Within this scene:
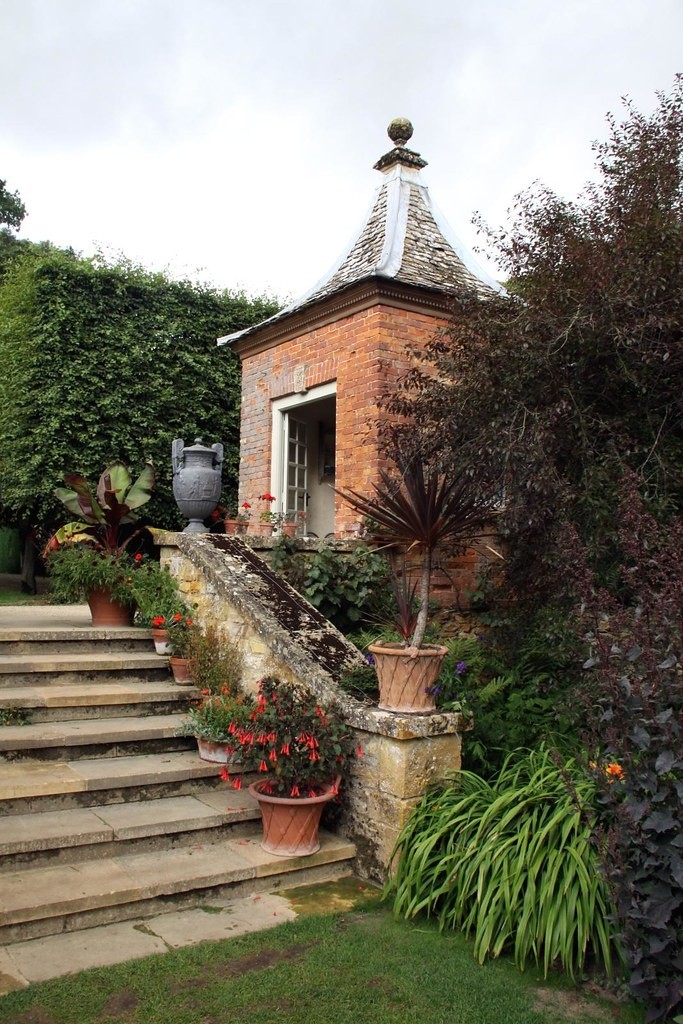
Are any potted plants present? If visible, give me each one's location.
[423,595,430,597]
[42,460,170,627]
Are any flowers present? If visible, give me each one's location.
[213,494,253,520]
[172,679,259,738]
[277,511,305,523]
[146,593,193,628]
[217,677,363,798]
[257,493,277,522]
[166,624,204,657]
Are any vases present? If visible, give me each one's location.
[259,522,275,536]
[327,438,502,713]
[151,628,175,656]
[283,523,296,537]
[195,736,245,763]
[247,778,339,857]
[225,520,250,535]
[170,657,195,685]
